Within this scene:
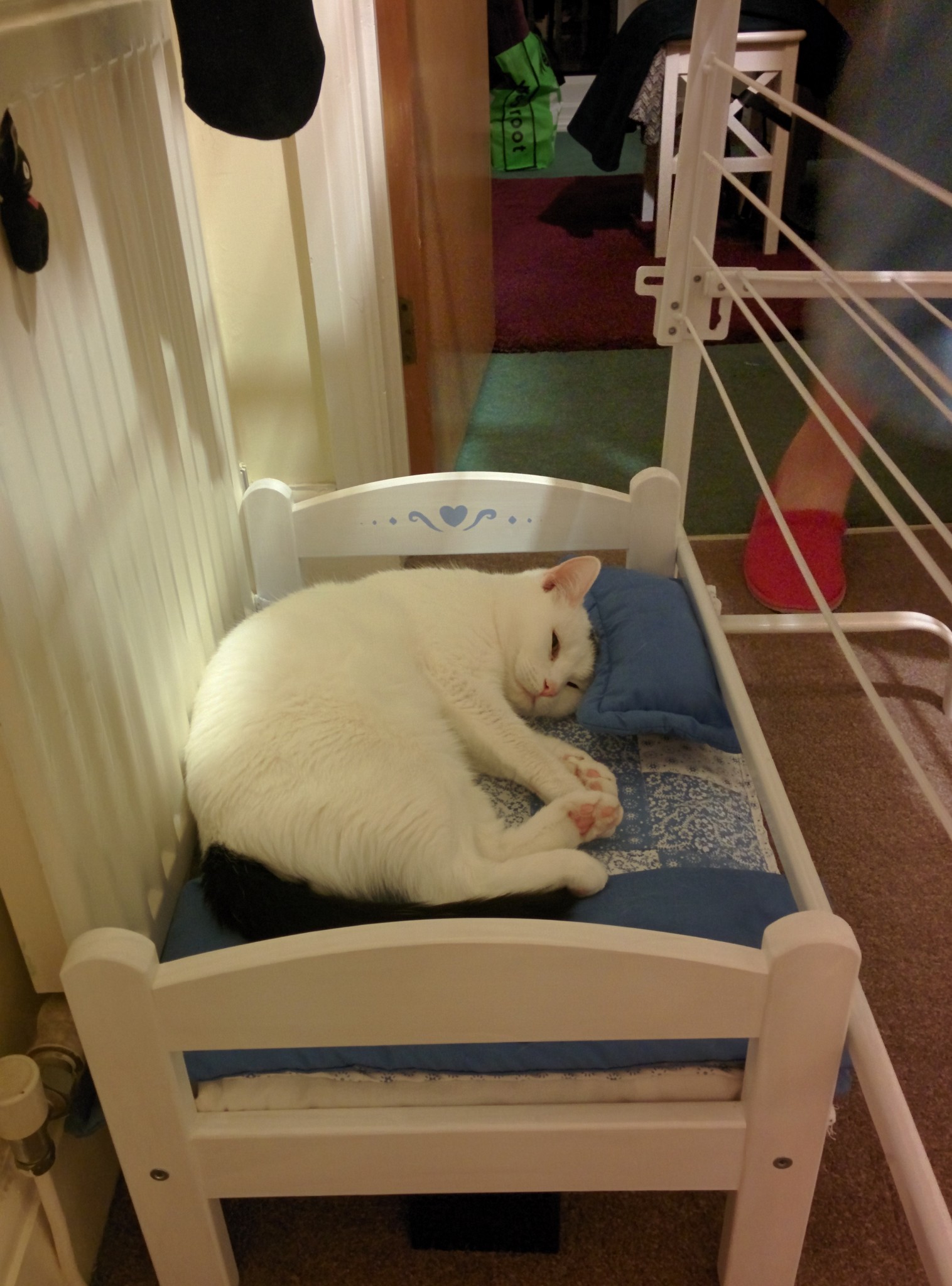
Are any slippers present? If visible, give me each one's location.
[742,485,845,615]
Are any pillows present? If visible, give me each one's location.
[579,556,743,758]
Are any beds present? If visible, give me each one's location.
[52,466,864,1286]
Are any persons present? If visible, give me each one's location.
[743,0,952,613]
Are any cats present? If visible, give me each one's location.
[186,555,624,944]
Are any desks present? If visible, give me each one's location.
[644,26,807,257]
